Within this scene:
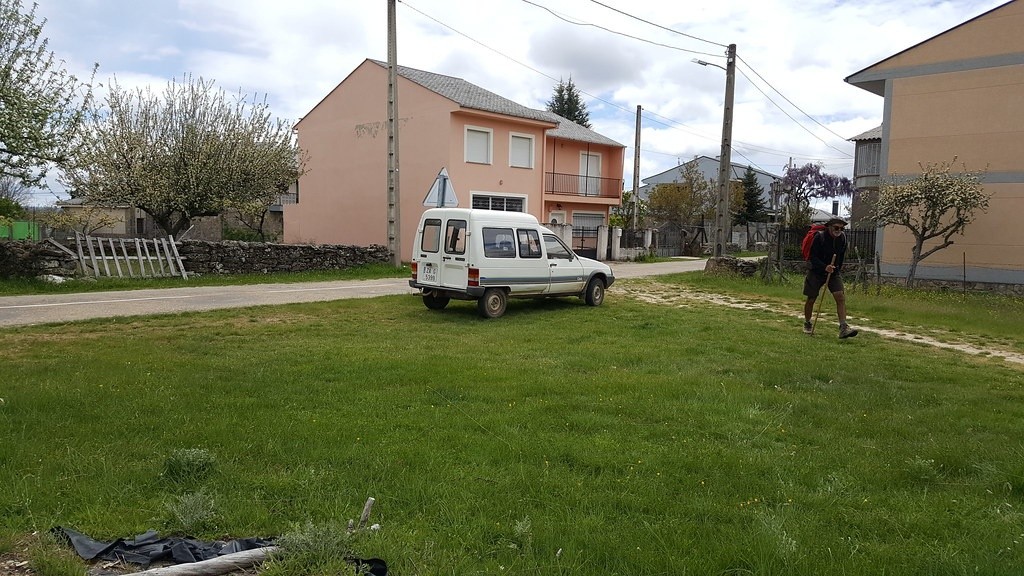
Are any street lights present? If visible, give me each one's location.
[690,44,736,256]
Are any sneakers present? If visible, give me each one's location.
[803,321,813,334]
[838,323,858,339]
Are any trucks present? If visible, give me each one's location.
[409,208,615,318]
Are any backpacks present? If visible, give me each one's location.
[801,225,826,268]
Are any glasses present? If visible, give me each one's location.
[834,226,845,231]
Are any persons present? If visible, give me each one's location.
[802,216,858,338]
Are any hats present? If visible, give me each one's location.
[824,216,848,227]
